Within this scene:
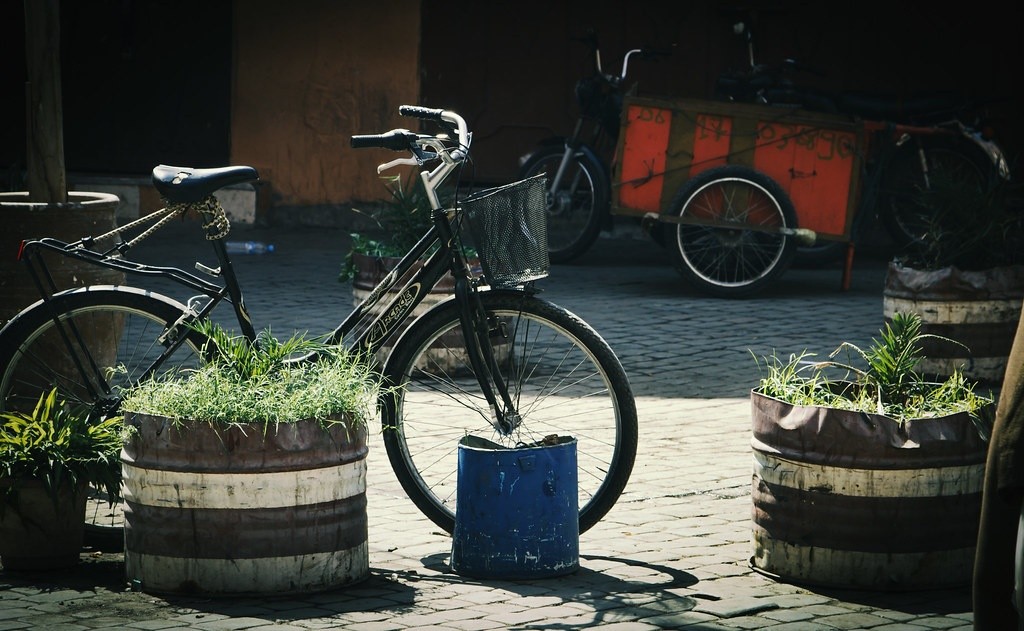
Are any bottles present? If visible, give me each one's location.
[225,241,275,256]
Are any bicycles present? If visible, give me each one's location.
[0,105,639,551]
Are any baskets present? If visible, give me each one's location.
[457,172,551,286]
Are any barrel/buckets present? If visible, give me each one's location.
[451,434,580,580]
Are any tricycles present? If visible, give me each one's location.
[509,41,871,298]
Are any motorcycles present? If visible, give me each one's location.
[713,21,1012,269]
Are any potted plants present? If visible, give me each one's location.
[746,309,999,595]
[0,385,124,573]
[882,150,1024,389]
[102,314,415,605]
[334,165,518,379]
